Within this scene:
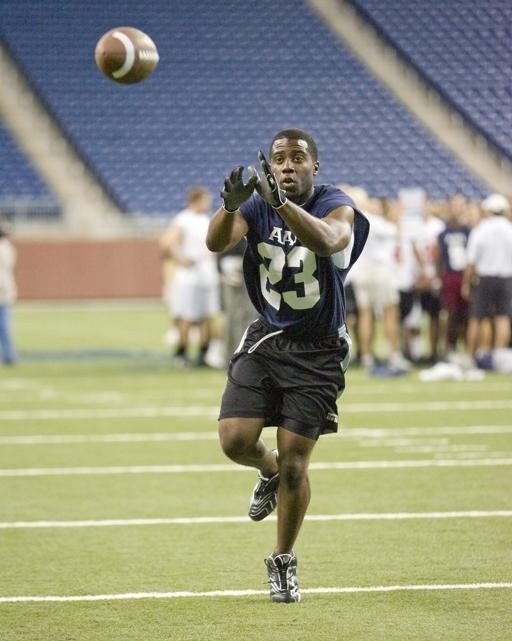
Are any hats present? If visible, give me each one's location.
[481,193,509,211]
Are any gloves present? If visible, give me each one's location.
[219,151,287,213]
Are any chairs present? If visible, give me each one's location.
[0,0,512,215]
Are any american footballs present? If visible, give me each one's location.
[95,26,160,85]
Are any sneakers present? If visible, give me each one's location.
[357,348,512,382]
[172,347,227,370]
[248,448,280,521]
[263,549,301,602]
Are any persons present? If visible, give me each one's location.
[205,128,369,603]
[334,182,512,379]
[0,216,21,364]
[156,188,259,370]
